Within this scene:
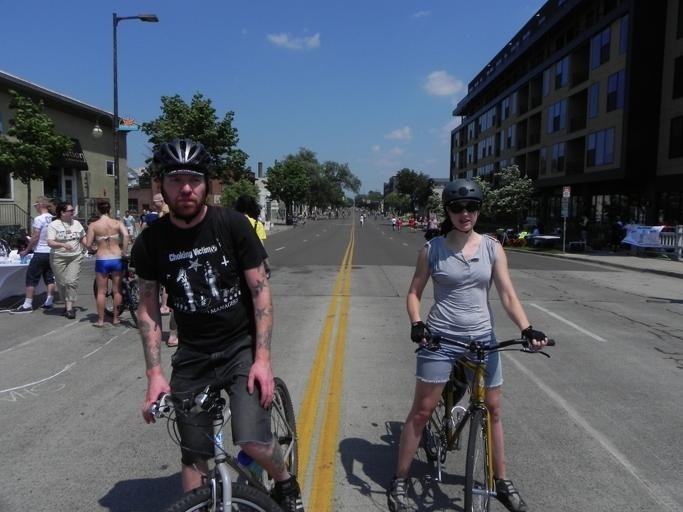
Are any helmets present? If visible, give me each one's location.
[153,138,210,177]
[442,179,482,206]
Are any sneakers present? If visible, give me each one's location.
[387,477,409,512]
[275,472,304,512]
[40,304,53,311]
[493,476,528,512]
[64,310,75,318]
[10,305,32,313]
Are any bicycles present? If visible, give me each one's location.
[146,376,298,512]
[93,257,140,330]
[416,324,554,512]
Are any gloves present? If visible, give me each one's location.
[411,321,430,343]
[521,325,546,348]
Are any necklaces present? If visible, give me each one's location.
[61,219,73,234]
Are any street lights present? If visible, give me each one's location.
[112,12,160,222]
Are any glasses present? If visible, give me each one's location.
[450,202,478,213]
[65,209,74,212]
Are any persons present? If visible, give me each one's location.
[363,213,368,230]
[139,209,147,230]
[528,228,532,234]
[610,215,635,238]
[46,196,63,220]
[84,199,129,327]
[392,216,396,231]
[153,192,169,217]
[359,213,364,231]
[579,215,590,250]
[146,208,158,227]
[537,222,545,234]
[46,204,95,320]
[408,216,440,232]
[388,179,548,512]
[527,225,540,248]
[122,210,135,243]
[8,195,56,313]
[130,137,306,512]
[235,194,266,248]
[398,218,402,231]
